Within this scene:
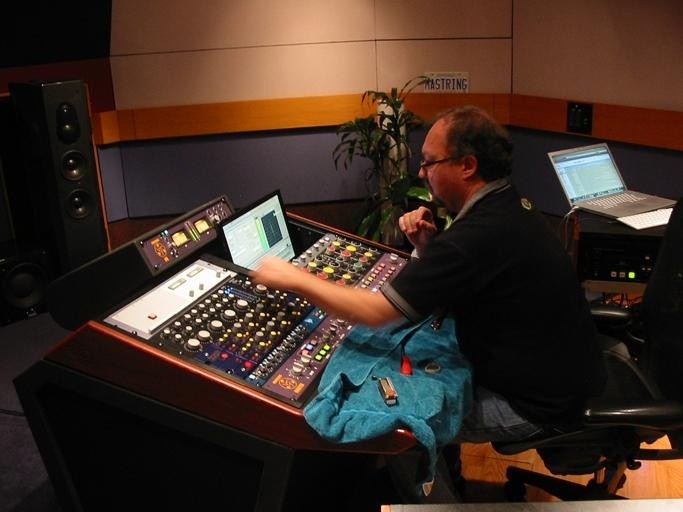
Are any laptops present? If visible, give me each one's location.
[547,142,678,221]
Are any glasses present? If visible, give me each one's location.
[420,156,464,167]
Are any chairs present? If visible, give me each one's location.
[502,198,683,501]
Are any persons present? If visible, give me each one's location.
[248,107,607,443]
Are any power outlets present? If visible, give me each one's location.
[566,101,593,136]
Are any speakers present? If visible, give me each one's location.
[7,78,112,279]
[0,249,58,326]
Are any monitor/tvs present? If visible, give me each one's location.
[218,188,297,275]
